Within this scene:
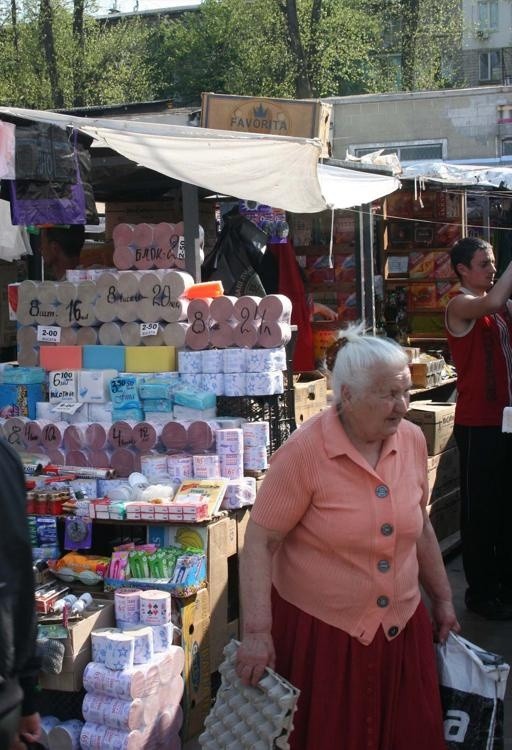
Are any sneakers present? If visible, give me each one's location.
[465,582,512,621]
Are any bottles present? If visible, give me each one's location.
[27,489,70,516]
[48,593,92,615]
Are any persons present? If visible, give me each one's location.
[233,321,462,750]
[440,234,512,621]
[34,223,86,281]
[312,301,339,322]
[0,436,45,749]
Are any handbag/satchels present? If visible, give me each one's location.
[434,629,511,749]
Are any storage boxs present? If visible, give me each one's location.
[201,91,332,163]
[36,598,116,692]
[105,200,217,246]
[162,590,214,749]
[422,489,464,541]
[407,403,459,455]
[421,447,463,505]
[293,370,329,430]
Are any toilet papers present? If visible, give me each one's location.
[33,587,186,749]
[0,222,292,519]
